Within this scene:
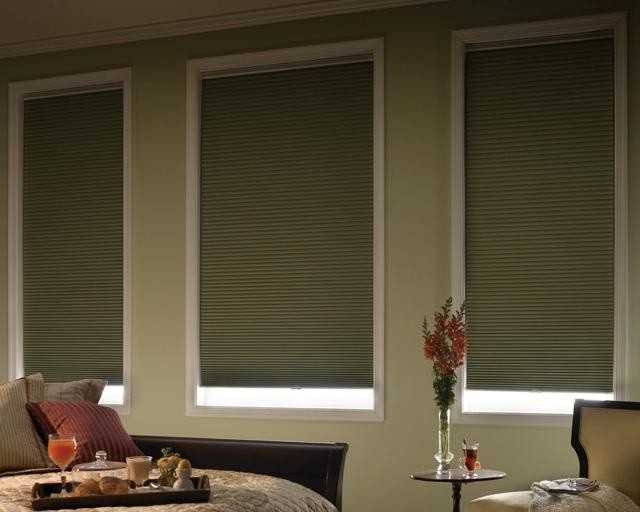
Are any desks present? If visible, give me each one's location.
[409,468,506,512]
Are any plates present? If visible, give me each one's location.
[30,475,210,511]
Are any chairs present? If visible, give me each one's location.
[469,397,640,512]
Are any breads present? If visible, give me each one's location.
[74,478,99,496]
[99,476,129,495]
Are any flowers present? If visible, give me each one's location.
[419,298,473,407]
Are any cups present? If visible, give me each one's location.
[463,444,478,477]
[48,434,76,497]
[127,456,153,490]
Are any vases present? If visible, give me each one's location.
[434,408,454,477]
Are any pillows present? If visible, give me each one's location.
[0,372,151,475]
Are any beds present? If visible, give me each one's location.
[0,435,349,512]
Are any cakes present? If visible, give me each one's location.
[156,447,182,487]
